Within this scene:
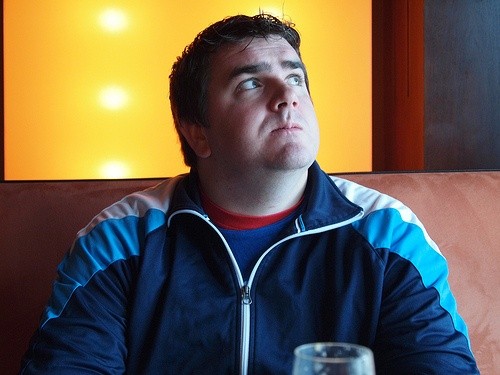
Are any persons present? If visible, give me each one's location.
[18,15,480,375]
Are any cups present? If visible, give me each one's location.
[292,342,376,375]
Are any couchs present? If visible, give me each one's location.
[0,168,500,375]
